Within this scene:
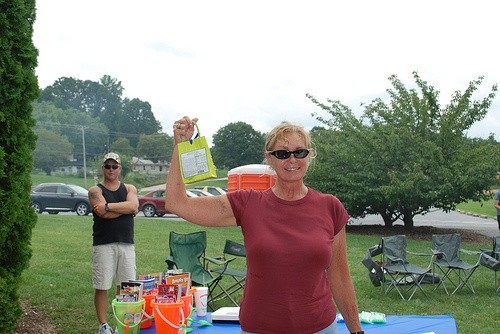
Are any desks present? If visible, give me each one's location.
[114,310,457,334]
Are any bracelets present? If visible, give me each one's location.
[351,331,364,334]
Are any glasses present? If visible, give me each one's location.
[105,164,119,170]
[269,148,310,160]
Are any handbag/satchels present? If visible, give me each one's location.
[177,121,217,184]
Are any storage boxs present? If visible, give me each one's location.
[211,306,240,324]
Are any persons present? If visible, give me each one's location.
[164,115,366,334]
[87,152,140,334]
[494,189,500,230]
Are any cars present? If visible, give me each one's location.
[138,189,199,218]
[188,185,229,196]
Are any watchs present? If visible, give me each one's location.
[104,203,110,212]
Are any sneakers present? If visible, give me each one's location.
[99,323,112,334]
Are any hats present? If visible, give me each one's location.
[103,153,120,164]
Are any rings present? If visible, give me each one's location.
[176,123,181,128]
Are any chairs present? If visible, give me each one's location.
[363,232,500,301]
[164,230,247,312]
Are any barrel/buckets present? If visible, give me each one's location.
[111,287,194,334]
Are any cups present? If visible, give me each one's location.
[194,287,209,317]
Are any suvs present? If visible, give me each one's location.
[29,182,94,216]
